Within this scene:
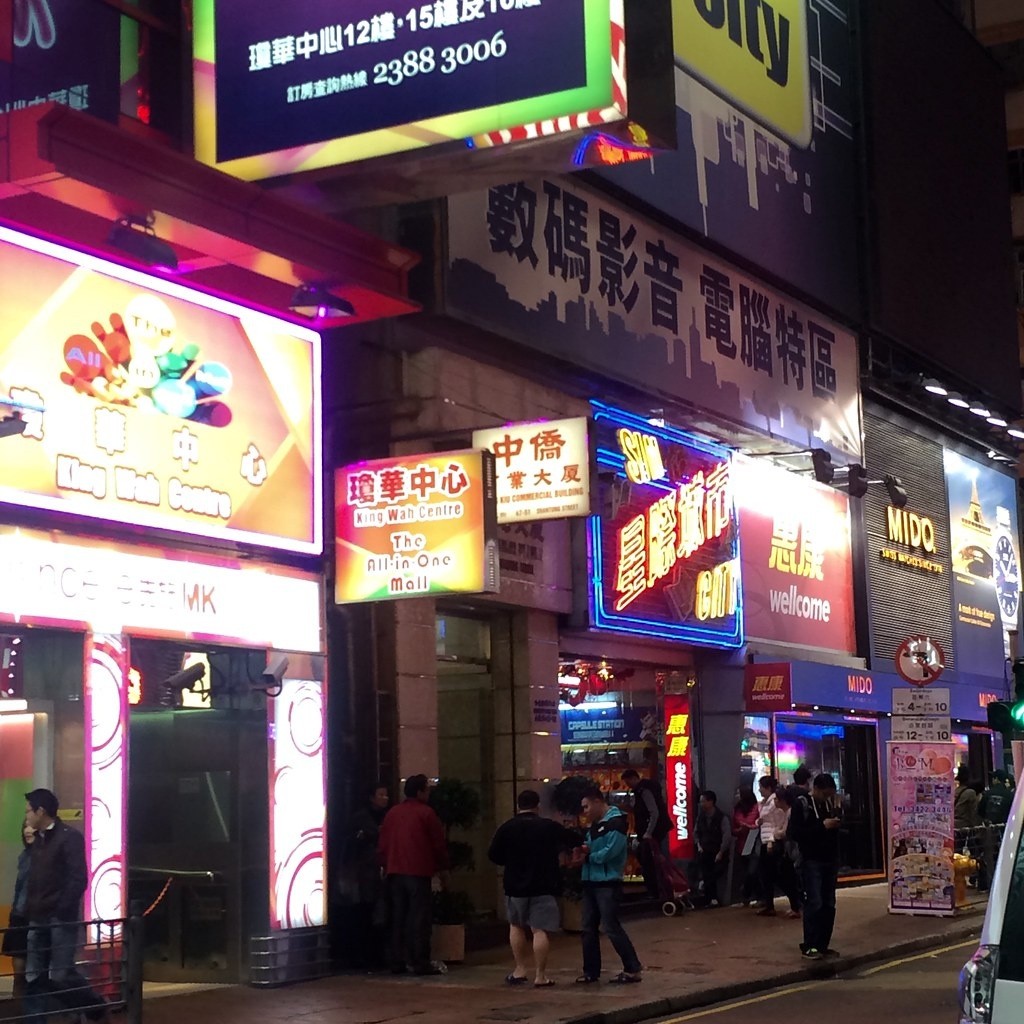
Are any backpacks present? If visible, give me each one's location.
[783,796,833,868]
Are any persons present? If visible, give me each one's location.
[618,769,814,920]
[337,782,399,976]
[488,790,576,987]
[572,789,644,985]
[789,774,849,962]
[955,766,1016,896]
[374,774,451,978]
[1,818,85,1024]
[23,788,112,1024]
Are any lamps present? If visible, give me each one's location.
[833,474,908,508]
[106,214,178,271]
[969,401,991,417]
[744,448,834,484]
[921,377,948,396]
[947,391,970,408]
[289,285,354,319]
[986,411,1008,427]
[1007,424,1024,439]
[791,463,869,499]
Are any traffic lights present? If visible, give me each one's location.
[1012,657,1024,699]
[986,700,1024,733]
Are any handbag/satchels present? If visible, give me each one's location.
[741,826,761,856]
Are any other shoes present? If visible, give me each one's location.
[415,965,442,975]
[783,910,801,918]
[752,900,765,909]
[817,948,839,958]
[730,901,745,908]
[755,907,776,917]
[705,899,719,909]
[696,881,704,896]
[801,948,823,959]
[392,962,408,973]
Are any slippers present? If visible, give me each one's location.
[609,972,642,983]
[505,971,529,984]
[534,978,556,987]
[576,974,599,984]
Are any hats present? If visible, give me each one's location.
[954,766,977,780]
[989,769,1006,780]
